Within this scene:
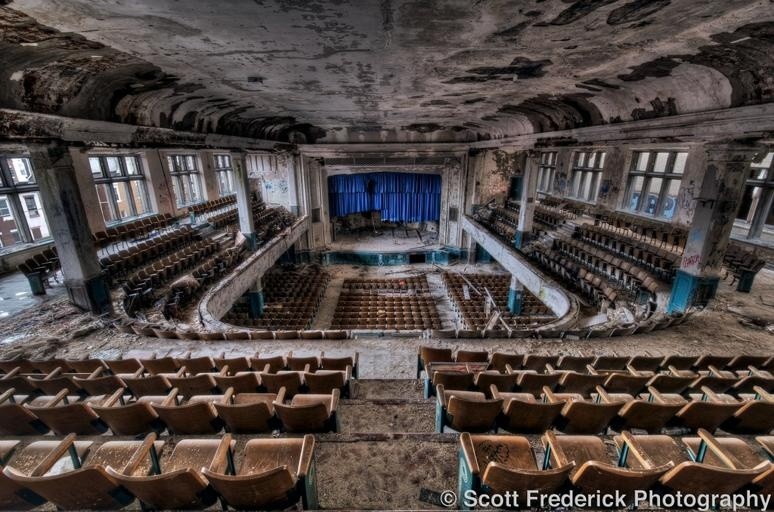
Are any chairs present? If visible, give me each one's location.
[2,322,354,511]
[19,181,294,321]
[411,321,774,510]
[294,258,488,328]
[490,185,763,319]
